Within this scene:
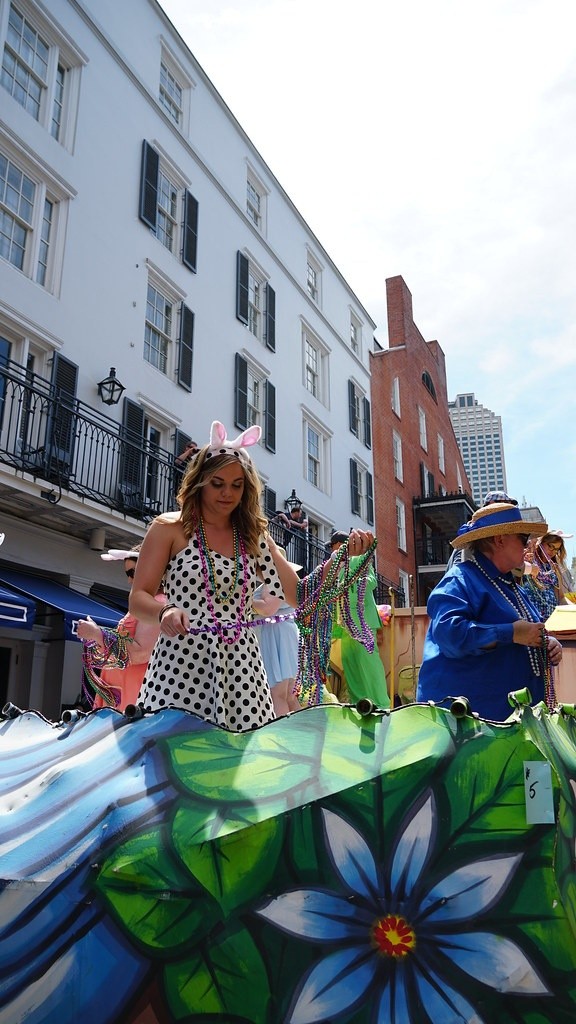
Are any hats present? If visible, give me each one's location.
[448,502,548,549]
[483,492,518,507]
[324,531,349,546]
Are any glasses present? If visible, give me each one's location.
[125,568,135,578]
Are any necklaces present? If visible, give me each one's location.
[497,575,512,585]
[532,543,561,589]
[191,509,250,645]
[470,555,542,677]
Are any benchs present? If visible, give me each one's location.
[117,484,163,518]
[16,438,76,484]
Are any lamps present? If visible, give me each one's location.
[283,489,303,512]
[99,365,127,406]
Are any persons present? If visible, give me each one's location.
[519,530,568,620]
[249,545,304,718]
[76,540,172,715]
[129,441,375,730]
[416,490,565,723]
[322,530,393,711]
[445,490,539,578]
